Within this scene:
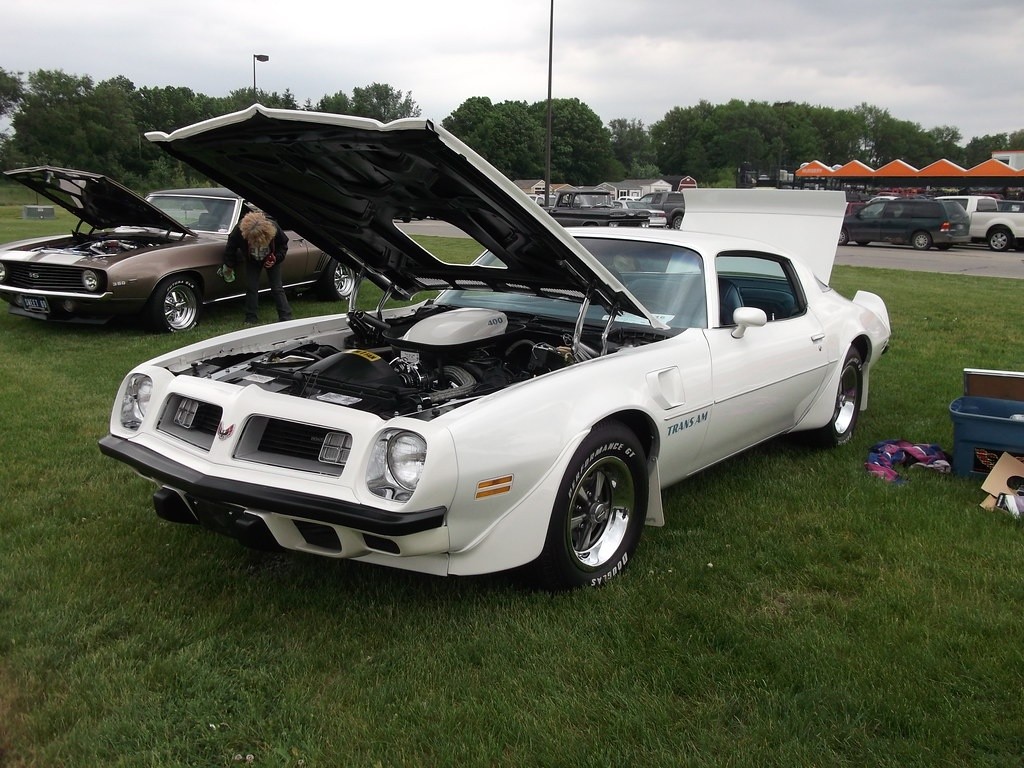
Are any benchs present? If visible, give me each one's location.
[740,287,796,321]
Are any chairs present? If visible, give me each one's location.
[679,275,744,325]
[189,213,220,231]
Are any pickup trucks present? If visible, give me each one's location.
[845,195,1024,252]
[526,185,685,231]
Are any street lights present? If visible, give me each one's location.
[254,54,270,94]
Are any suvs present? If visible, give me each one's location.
[837,199,971,251]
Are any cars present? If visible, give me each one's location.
[0,165,357,338]
[97,102,891,591]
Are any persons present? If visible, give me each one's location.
[226,211,293,320]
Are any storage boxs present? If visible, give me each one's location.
[949,396,1024,478]
